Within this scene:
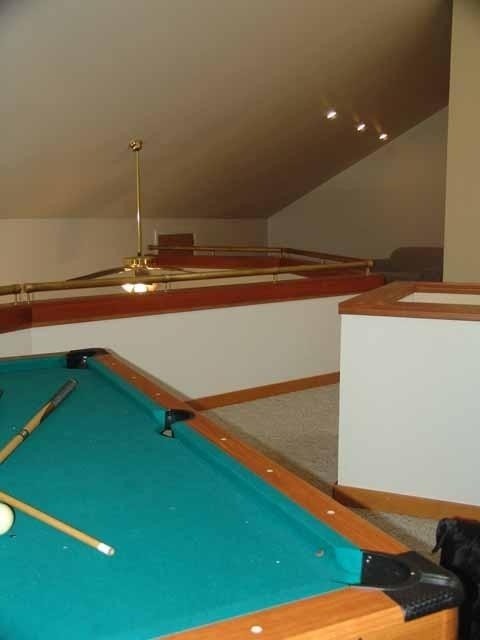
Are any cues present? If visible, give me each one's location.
[0,492,115,556]
[0,378,78,463]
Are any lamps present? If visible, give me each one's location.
[118,135,161,294]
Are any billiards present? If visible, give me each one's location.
[1,504,15,534]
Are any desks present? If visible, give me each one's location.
[0,349,463,640]
